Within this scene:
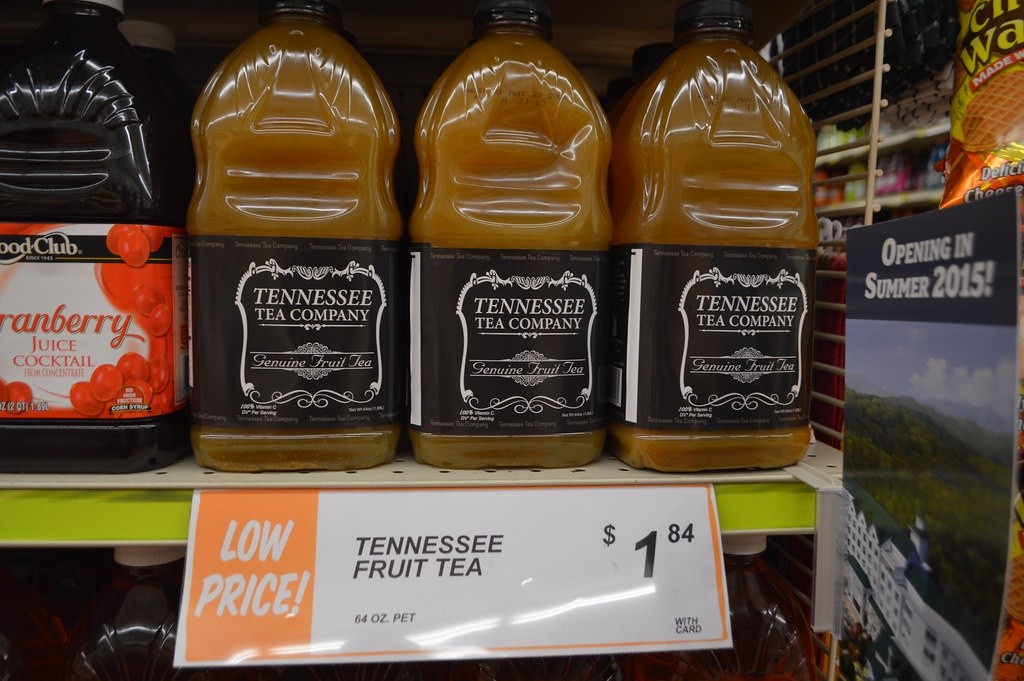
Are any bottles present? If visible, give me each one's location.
[0,545,822,681]
[0,0,816,474]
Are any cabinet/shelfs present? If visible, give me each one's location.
[812,117,958,217]
[0,0,897,681]
